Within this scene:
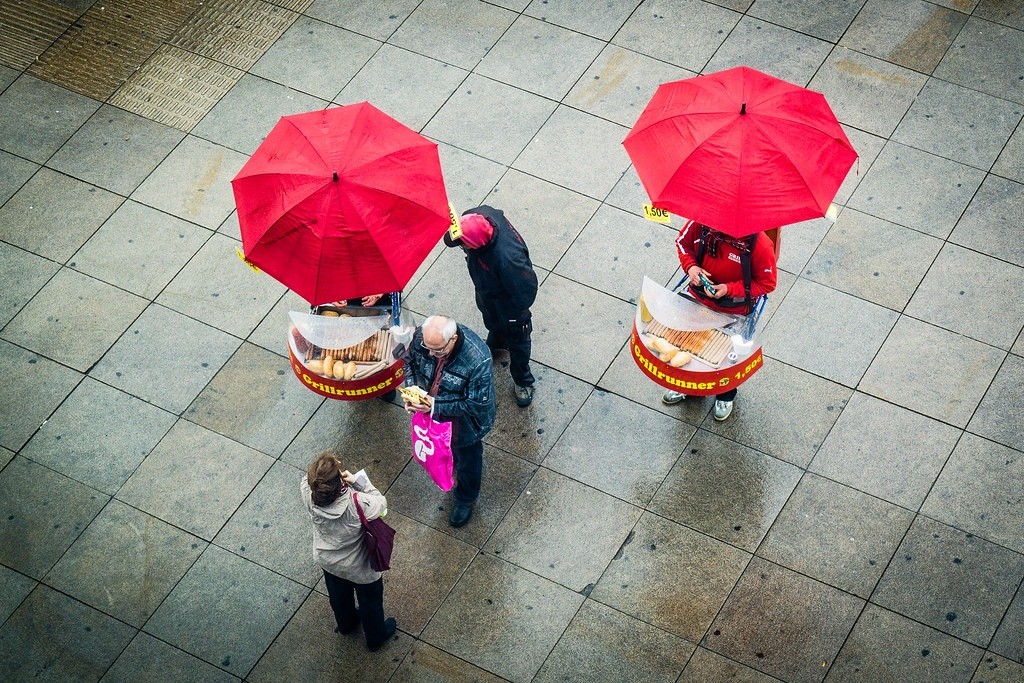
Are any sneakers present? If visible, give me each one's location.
[662,390,687,404]
[514,383,533,407]
[713,398,733,421]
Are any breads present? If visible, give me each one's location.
[321,311,352,318]
[645,336,691,368]
[307,355,357,380]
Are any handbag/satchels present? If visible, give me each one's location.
[353,493,396,572]
[411,397,454,492]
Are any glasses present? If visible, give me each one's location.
[420,333,456,354]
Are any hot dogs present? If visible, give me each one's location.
[398,386,431,407]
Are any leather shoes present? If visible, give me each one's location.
[450,500,473,528]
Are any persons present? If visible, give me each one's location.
[310,294,396,401]
[402,315,496,527]
[662,220,778,421]
[301,453,397,652]
[444,206,539,405]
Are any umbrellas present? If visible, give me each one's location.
[232,101,453,307]
[623,66,857,238]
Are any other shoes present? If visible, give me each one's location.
[367,617,397,652]
[340,607,361,634]
[384,389,396,402]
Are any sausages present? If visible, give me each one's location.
[351,360,386,381]
[643,318,732,364]
[305,328,391,364]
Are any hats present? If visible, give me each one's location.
[444,231,464,247]
